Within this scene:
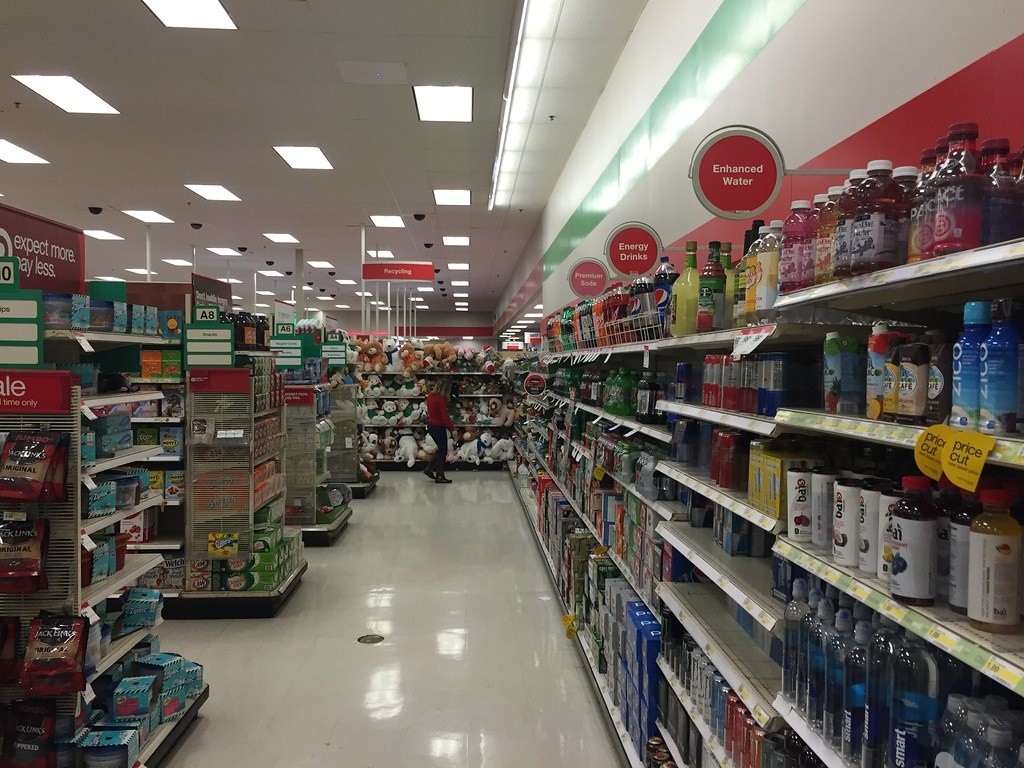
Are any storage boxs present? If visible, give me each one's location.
[81,431,95,462]
[44,362,101,395]
[160,427,184,456]
[162,384,185,417]
[161,350,182,378]
[537,429,714,762]
[81,599,111,677]
[81,480,117,519]
[142,350,162,378]
[750,449,825,522]
[42,293,91,332]
[120,578,137,590]
[81,540,110,588]
[165,470,184,499]
[316,484,352,524]
[91,475,142,510]
[149,470,165,491]
[770,551,807,607]
[143,306,158,335]
[88,533,132,577]
[90,301,128,333]
[132,385,159,417]
[195,459,286,515]
[120,505,159,543]
[55,633,204,768]
[81,415,131,435]
[137,551,163,589]
[137,429,158,445]
[95,430,133,454]
[686,488,776,557]
[126,304,145,334]
[120,588,165,627]
[190,495,305,592]
[96,467,149,500]
[725,593,783,667]
[163,551,185,589]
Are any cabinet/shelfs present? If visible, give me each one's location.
[505,308,663,768]
[284,382,353,547]
[0,332,210,768]
[178,349,309,619]
[328,362,380,499]
[106,332,184,621]
[654,323,791,768]
[770,236,1024,768]
[360,371,503,463]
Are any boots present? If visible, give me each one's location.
[423,451,436,479]
[435,454,453,483]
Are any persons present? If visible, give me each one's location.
[423,378,458,483]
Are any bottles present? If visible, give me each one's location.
[545,118,1024,768]
[218,311,271,352]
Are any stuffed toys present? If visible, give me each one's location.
[351,338,517,468]
